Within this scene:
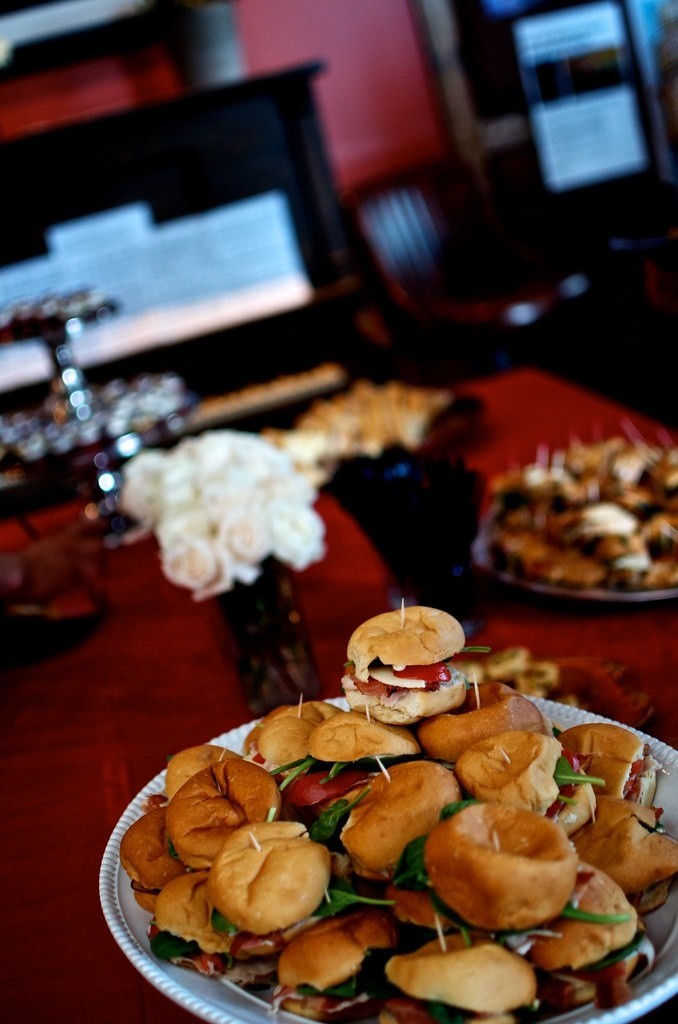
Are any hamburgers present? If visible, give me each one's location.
[119,606,678,1024]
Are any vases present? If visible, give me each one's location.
[213,556,327,718]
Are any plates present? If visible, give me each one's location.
[472,506,678,601]
[100,694,678,1024]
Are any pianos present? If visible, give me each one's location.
[1,57,447,525]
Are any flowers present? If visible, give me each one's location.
[117,429,327,604]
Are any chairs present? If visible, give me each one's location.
[337,160,570,331]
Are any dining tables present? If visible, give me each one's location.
[0,368,678,1024]
